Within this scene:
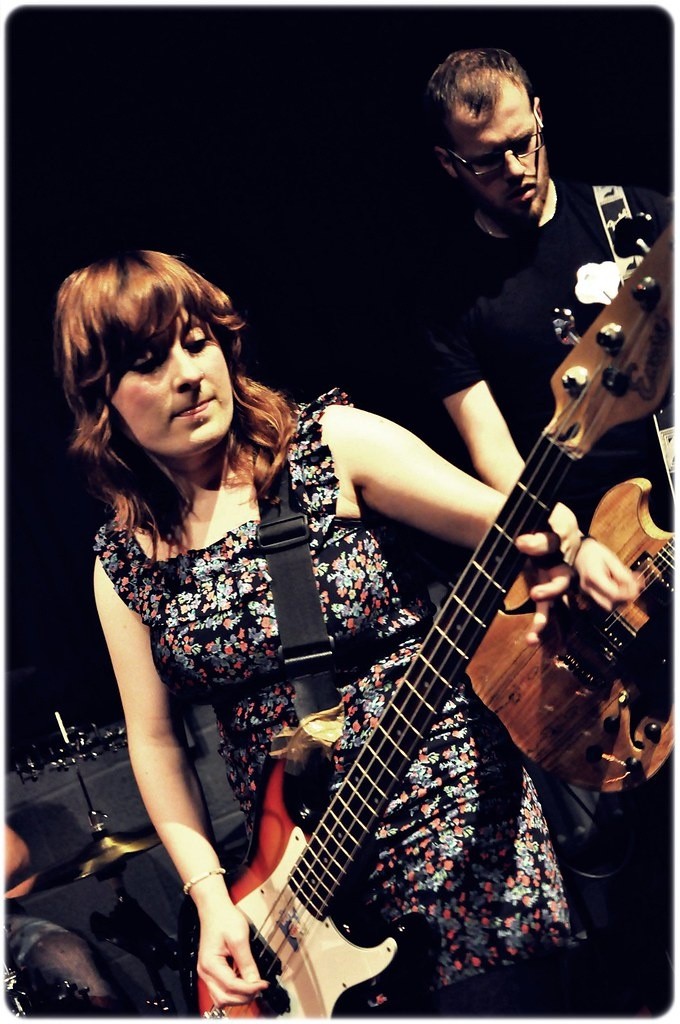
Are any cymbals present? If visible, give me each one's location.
[5,823,161,905]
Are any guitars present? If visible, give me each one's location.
[200,212,674,1019]
[468,475,674,798]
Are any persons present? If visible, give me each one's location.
[50,241,589,1019]
[417,40,673,602]
[4,817,114,1014]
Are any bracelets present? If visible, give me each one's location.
[181,866,227,895]
[560,533,599,567]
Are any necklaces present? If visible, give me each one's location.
[473,209,495,238]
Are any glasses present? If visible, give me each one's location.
[448,108,546,177]
